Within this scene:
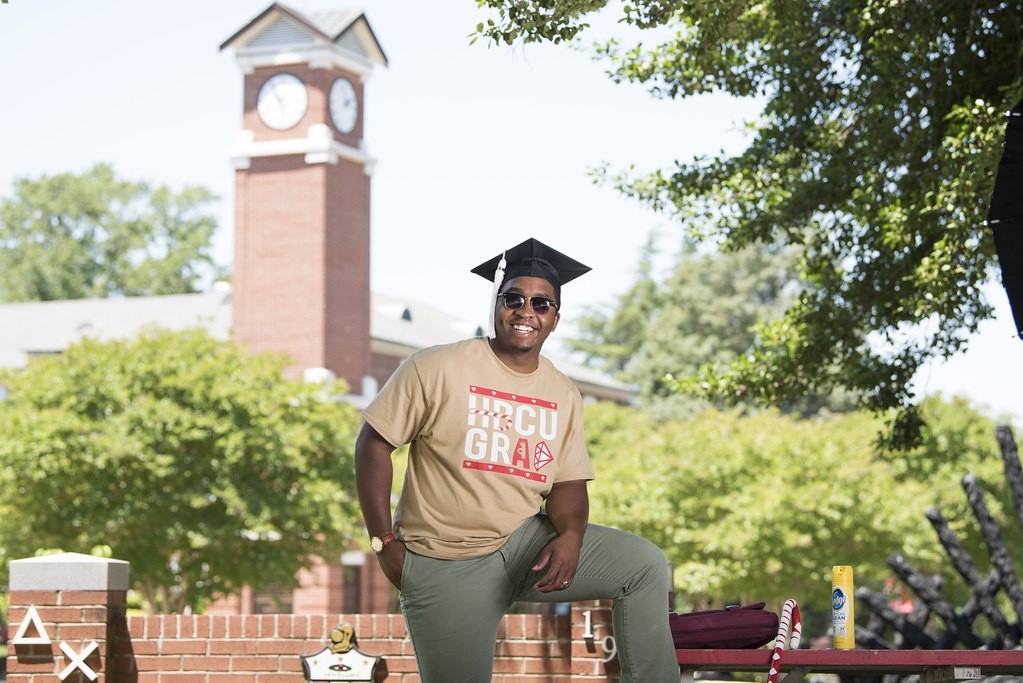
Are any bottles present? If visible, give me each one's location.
[831,565,855,650]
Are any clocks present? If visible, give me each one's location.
[255,72,310,131]
[327,74,359,135]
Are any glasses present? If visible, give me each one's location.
[497,292,558,315]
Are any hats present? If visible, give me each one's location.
[470,237,592,338]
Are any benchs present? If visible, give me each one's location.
[673,645,1023,683]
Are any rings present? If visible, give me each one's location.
[561,580,570,589]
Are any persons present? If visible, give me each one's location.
[353,237,679,683]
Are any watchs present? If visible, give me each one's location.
[369,534,393,552]
